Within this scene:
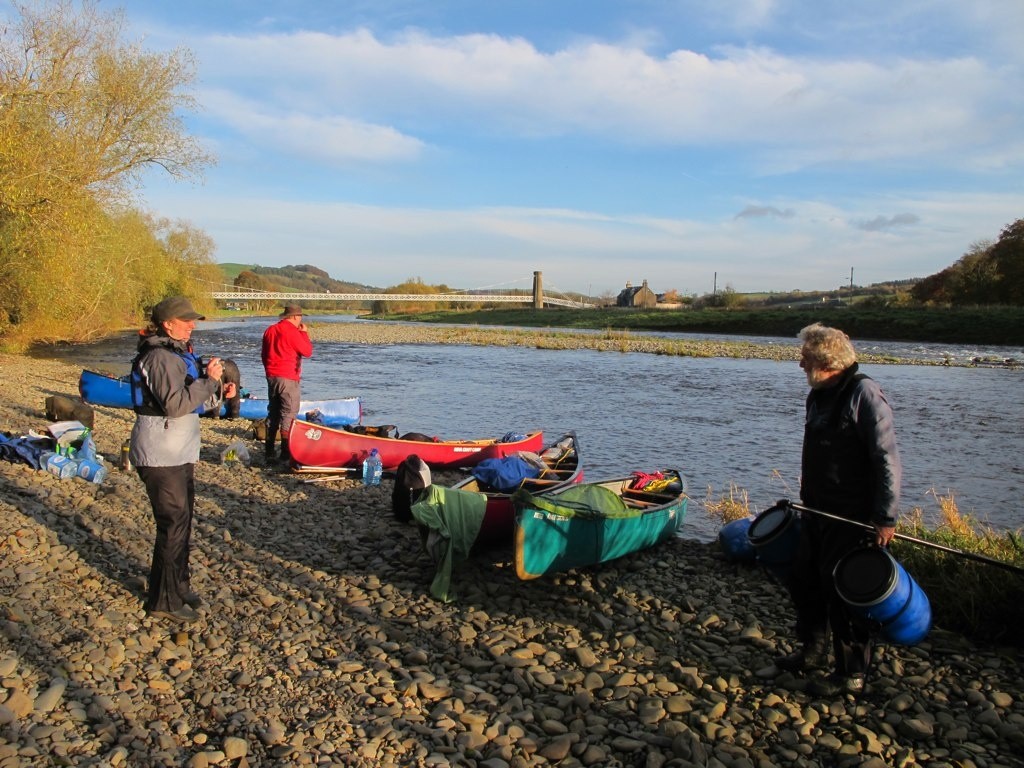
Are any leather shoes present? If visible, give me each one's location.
[148,605,198,624]
[179,591,201,607]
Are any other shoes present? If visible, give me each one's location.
[807,672,864,698]
[773,648,828,673]
[279,459,291,465]
[265,456,278,465]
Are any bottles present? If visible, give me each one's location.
[36,452,77,479]
[362,448,383,484]
[120,439,135,472]
[69,458,108,483]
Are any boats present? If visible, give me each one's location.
[407,429,584,552]
[510,468,688,581]
[285,416,545,473]
[78,369,364,430]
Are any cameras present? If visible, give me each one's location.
[215,360,225,371]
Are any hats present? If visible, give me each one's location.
[151,297,206,327]
[278,301,308,317]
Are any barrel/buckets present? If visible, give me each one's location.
[718,501,802,604]
[831,542,931,646]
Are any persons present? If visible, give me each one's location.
[128,296,236,624]
[786,321,899,698]
[211,359,240,419]
[261,301,315,462]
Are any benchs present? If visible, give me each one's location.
[541,469,574,473]
[621,496,659,508]
[522,478,562,485]
